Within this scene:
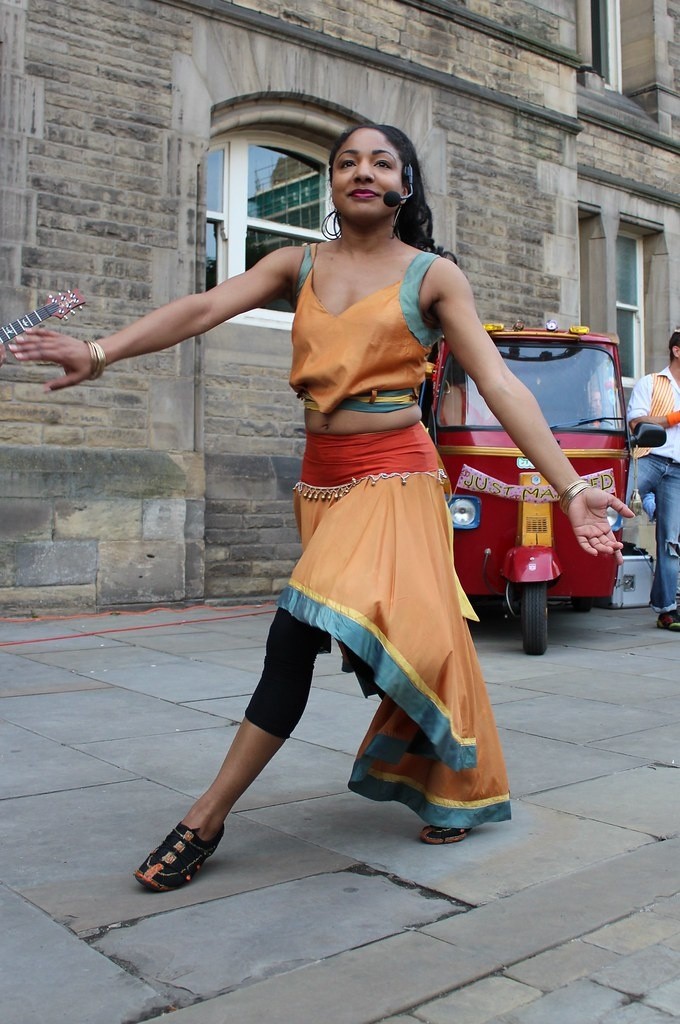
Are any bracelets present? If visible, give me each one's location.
[559,479,592,517]
[83,340,107,380]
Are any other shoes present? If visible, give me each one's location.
[137,817,224,892]
[420,821,481,843]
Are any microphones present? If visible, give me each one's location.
[384,164,414,208]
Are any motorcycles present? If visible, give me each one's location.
[418,319,630,657]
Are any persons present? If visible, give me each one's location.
[626,326,680,632]
[6,120,638,896]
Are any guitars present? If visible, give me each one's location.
[1,288,85,365]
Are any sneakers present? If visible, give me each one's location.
[655,610,680,631]
